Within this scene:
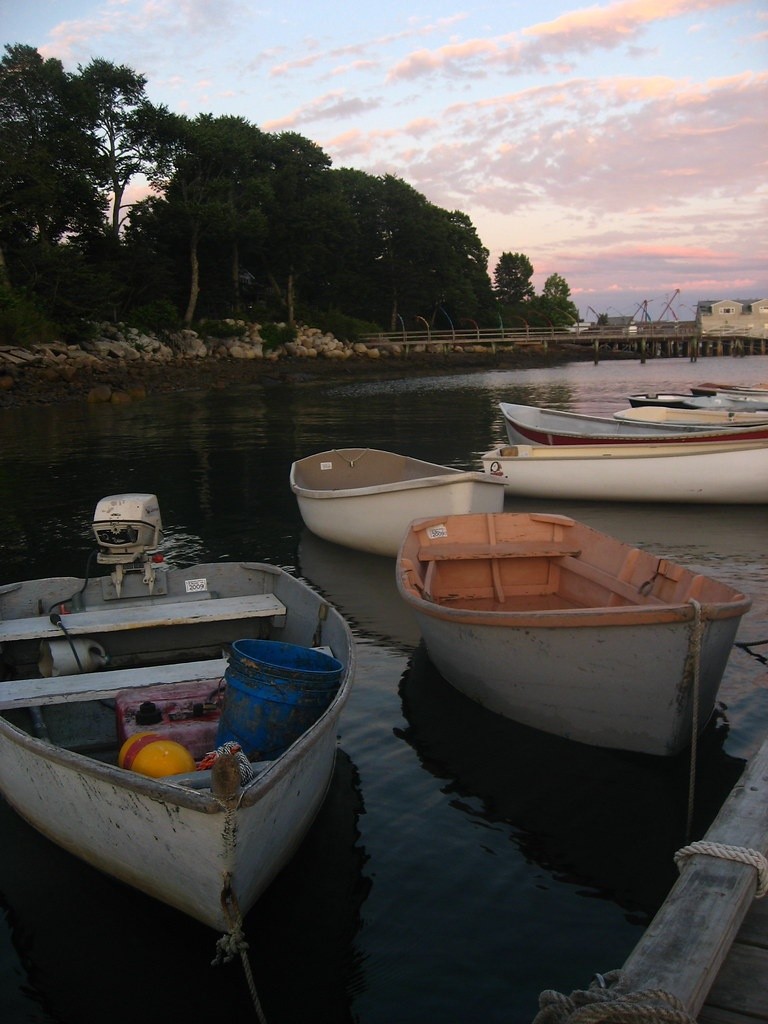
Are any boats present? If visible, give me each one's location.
[499,402,768,441]
[613,405,768,428]
[625,391,768,412]
[1,494,360,937]
[690,383,768,398]
[289,448,511,558]
[478,437,768,508]
[395,510,753,756]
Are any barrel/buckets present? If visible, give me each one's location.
[212,638,342,764]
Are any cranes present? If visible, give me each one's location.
[585,288,697,327]
